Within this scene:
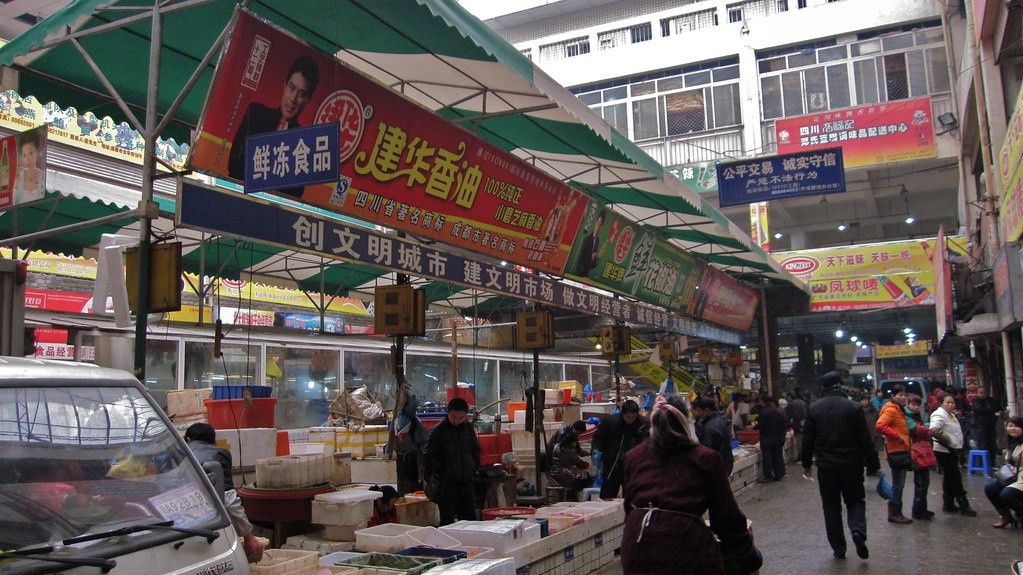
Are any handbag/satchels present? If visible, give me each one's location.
[540,453,553,472]
[888,450,912,471]
[877,477,893,499]
[996,464,1017,487]
[911,441,937,469]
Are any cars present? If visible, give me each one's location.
[0,355,252,575]
[877,379,929,423]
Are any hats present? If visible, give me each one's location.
[821,371,845,386]
[934,388,948,402]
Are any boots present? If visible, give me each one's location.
[887,501,913,524]
[942,495,959,511]
[992,507,1014,528]
[956,491,977,517]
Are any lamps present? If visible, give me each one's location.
[902,325,917,347]
[836,324,868,349]
[937,112,957,127]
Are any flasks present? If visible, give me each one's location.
[494,413,501,434]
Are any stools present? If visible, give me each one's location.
[967,450,992,477]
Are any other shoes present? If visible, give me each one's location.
[912,510,934,520]
[853,532,868,559]
[758,475,770,482]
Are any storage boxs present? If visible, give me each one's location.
[142,378,759,575]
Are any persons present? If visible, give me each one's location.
[424,398,481,526]
[693,272,714,317]
[708,384,1023,528]
[621,394,762,575]
[984,416,1023,528]
[691,397,734,477]
[22,409,130,512]
[801,371,880,560]
[591,400,652,499]
[545,420,593,502]
[12,130,45,205]
[367,484,401,529]
[227,56,319,198]
[574,209,606,277]
[160,423,259,554]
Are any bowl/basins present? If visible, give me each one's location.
[736,429,760,442]
[481,507,537,520]
